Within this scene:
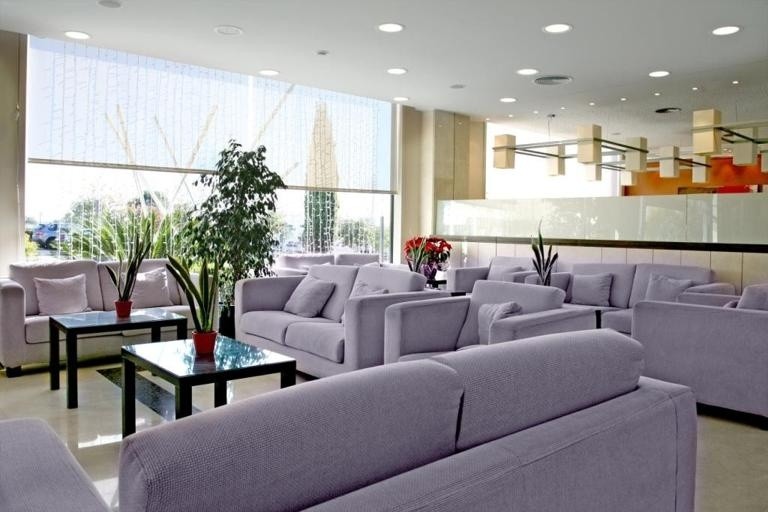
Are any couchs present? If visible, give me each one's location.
[1,259,220,379]
[117,326,696,511]
[233,254,767,419]
[0,417,109,511]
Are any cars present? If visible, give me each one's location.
[31,222,93,250]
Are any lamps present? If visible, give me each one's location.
[491,107,767,185]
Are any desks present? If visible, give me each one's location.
[49,308,187,410]
[120,336,297,439]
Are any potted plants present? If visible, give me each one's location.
[105,219,154,318]
[173,137,290,353]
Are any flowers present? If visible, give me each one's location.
[404,235,453,279]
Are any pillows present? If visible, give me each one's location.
[478,301,523,345]
[488,265,521,281]
[120,267,174,310]
[341,279,389,327]
[284,274,335,318]
[571,272,611,307]
[32,274,92,316]
[644,272,692,302]
